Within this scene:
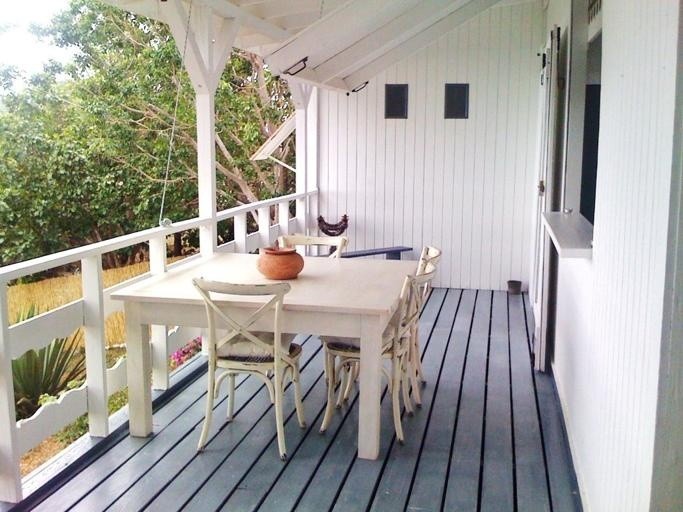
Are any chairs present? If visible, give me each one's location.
[323,245,441,411]
[319,256,437,443]
[193,279,307,460]
[279,235,347,258]
